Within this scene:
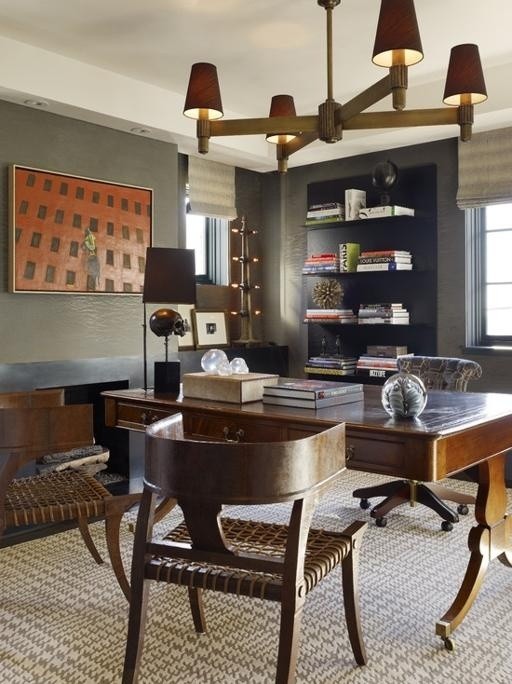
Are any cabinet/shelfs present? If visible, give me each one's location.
[300,213,430,387]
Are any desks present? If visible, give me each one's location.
[100,371,512,651]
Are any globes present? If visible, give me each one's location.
[369,160,399,206]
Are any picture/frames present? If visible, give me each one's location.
[6,163,155,299]
[177,302,231,352]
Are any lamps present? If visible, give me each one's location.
[142,246,195,395]
[183,0,488,176]
[231,215,264,347]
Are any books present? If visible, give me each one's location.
[181,372,365,409]
[301,189,415,376]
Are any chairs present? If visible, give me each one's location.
[119,410,369,684]
[0,387,141,605]
[353,354,486,533]
